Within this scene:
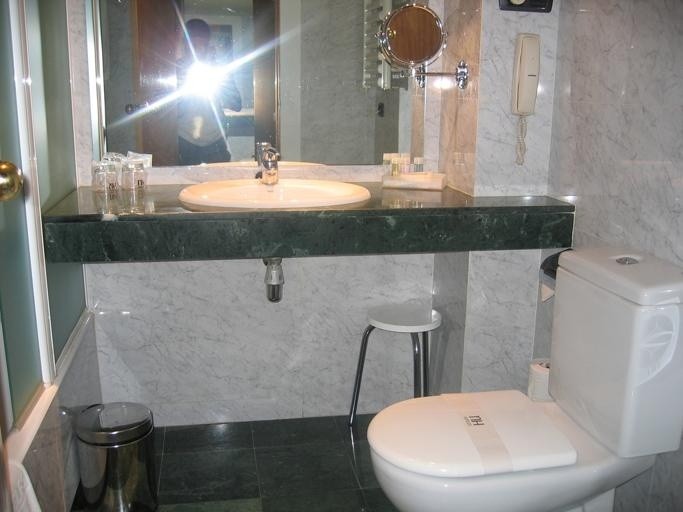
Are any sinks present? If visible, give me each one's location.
[177,178,371,208]
[198,161,326,167]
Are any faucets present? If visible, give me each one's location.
[252,142,272,166]
[257,147,280,186]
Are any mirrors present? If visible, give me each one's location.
[378,4,468,89]
[129,0,281,169]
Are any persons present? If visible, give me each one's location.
[175,18,243,166]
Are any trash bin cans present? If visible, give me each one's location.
[63,402,159,512]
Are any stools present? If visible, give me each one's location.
[347,302,443,426]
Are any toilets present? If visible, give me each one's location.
[365,244,682,511]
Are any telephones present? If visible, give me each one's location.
[511,32,541,115]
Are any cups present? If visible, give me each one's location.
[94,193,119,216]
[121,162,145,192]
[92,164,117,191]
[121,193,146,215]
